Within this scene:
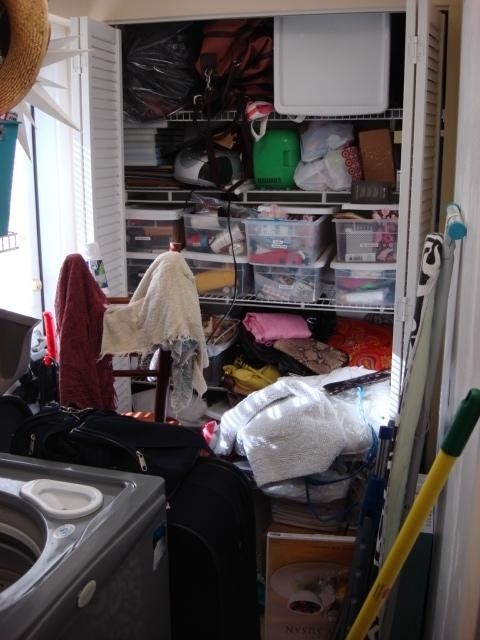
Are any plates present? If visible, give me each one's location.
[271,560,349,601]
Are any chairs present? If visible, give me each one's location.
[55,252,187,425]
[1,309,42,397]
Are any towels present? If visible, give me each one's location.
[54,254,119,414]
[236,380,346,486]
[97,252,212,419]
[212,381,293,456]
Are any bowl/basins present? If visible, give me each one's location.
[286,589,326,617]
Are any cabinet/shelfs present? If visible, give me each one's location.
[70,0,449,415]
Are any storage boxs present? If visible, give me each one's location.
[198,327,239,386]
[123,206,402,309]
[1,120,23,236]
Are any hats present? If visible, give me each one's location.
[0,1,50,115]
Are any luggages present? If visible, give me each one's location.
[15,399,261,639]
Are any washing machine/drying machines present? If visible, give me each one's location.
[0,452,174,640]
